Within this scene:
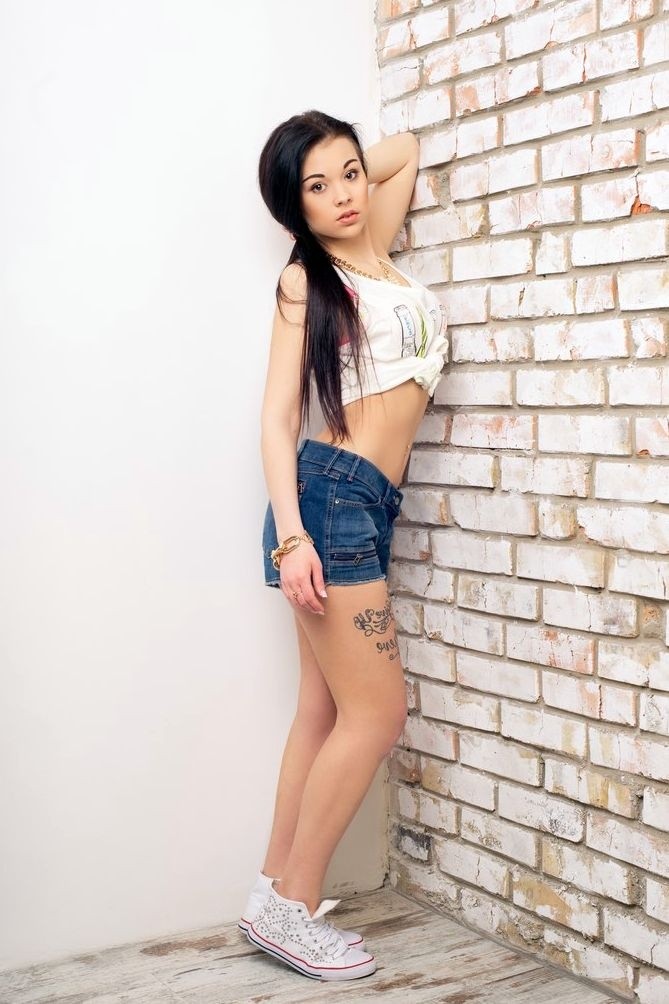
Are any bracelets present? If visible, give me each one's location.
[270,530,314,570]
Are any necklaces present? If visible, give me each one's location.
[327,252,393,283]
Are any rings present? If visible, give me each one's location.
[292,591,302,598]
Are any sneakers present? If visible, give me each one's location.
[246,879,377,981]
[238,869,366,952]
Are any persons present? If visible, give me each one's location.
[238,110,450,982]
